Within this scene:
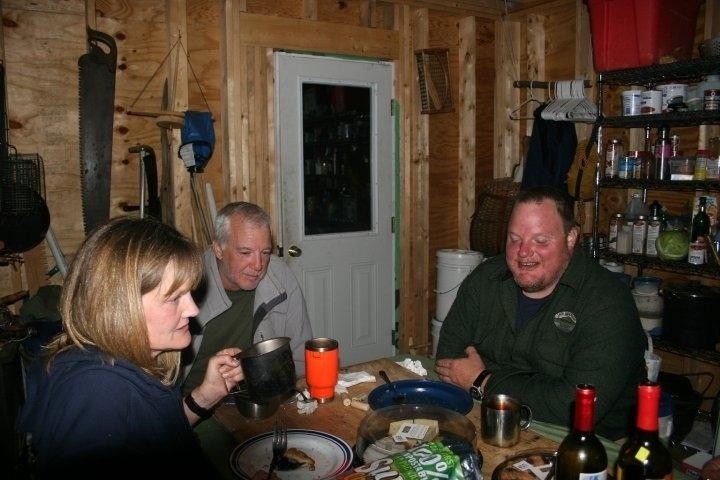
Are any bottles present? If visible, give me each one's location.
[701,75,720,89]
[606,191,718,270]
[603,122,720,184]
[555,383,609,480]
[631,278,663,336]
[613,380,674,480]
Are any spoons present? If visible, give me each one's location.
[379,369,408,405]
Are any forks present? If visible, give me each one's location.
[264,415,289,480]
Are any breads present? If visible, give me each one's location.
[389,418,439,448]
[252,469,279,480]
[284,448,315,471]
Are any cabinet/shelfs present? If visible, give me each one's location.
[591,56,719,480]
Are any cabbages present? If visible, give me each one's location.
[655,230,690,262]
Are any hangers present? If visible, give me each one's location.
[510,79,605,123]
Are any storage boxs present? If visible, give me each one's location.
[584,1,703,75]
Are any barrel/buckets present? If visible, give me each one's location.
[434,249,485,322]
[430,319,444,358]
[624,194,651,222]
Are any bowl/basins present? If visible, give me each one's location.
[357,405,478,470]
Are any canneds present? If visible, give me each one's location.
[667,135,680,158]
[703,89,720,110]
[622,84,688,116]
[688,240,706,265]
[619,157,655,179]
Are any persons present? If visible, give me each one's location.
[174,202,313,382]
[436,187,649,443]
[19,215,247,480]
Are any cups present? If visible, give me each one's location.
[222,338,297,405]
[305,337,338,405]
[480,394,532,447]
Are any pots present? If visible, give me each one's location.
[664,279,720,349]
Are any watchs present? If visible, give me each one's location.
[468,369,491,400]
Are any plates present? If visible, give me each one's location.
[229,430,353,480]
[369,379,474,416]
[492,453,556,480]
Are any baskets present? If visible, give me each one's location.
[467,172,527,259]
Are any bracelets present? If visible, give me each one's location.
[182,394,213,418]
